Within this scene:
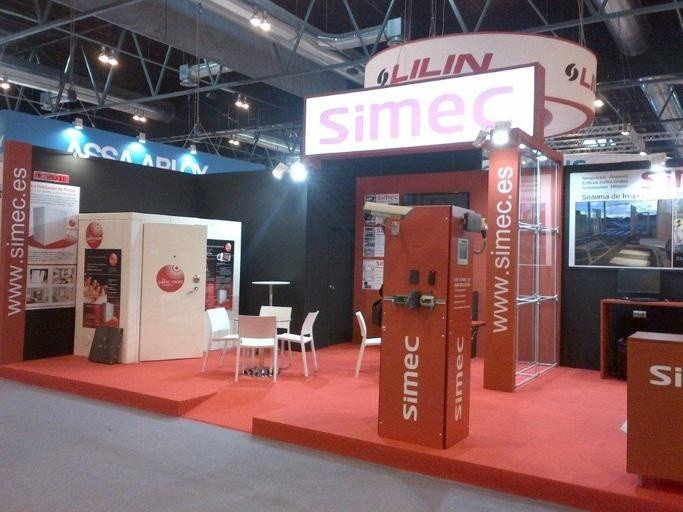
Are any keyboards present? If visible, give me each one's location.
[630,298,659,301]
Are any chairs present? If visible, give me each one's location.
[277,311,319,376]
[235,317,279,382]
[355,311,381,378]
[202,307,242,374]
[259,306,292,366]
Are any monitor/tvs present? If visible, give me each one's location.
[617,268,661,300]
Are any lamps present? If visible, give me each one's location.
[0,0,683,182]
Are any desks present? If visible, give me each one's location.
[254,281,291,352]
[599,298,683,379]
[233,317,289,376]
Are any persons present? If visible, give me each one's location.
[26,268,76,304]
[84,274,93,297]
[90,279,98,298]
[95,286,101,298]
[100,288,106,296]
